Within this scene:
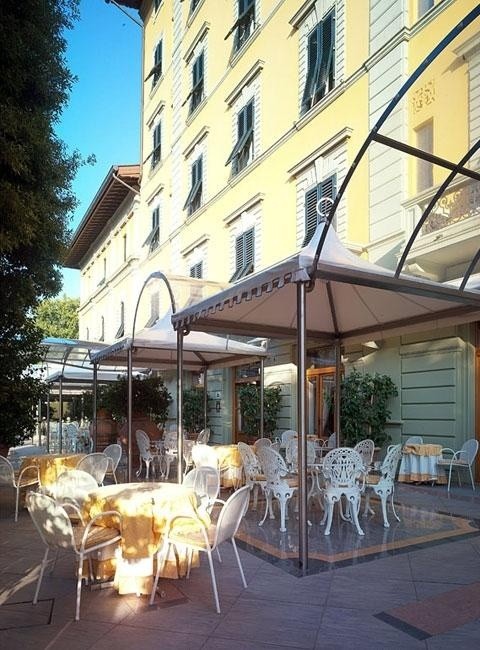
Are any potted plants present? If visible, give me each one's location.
[345,369,398,462]
[181,387,213,440]
[106,373,173,468]
[69,383,117,453]
[240,383,283,446]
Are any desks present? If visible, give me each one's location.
[19,453,86,508]
[399,444,447,488]
[211,444,256,491]
[75,483,210,599]
[307,461,356,522]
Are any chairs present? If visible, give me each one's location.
[91,444,122,486]
[52,469,98,510]
[75,453,109,487]
[183,441,194,475]
[25,492,123,622]
[135,429,165,480]
[182,466,223,580]
[351,442,403,528]
[431,438,479,492]
[353,440,375,516]
[286,438,326,514]
[238,442,291,520]
[0,456,41,522]
[162,432,185,480]
[319,447,366,536]
[387,436,423,460]
[257,446,312,532]
[254,438,271,447]
[196,428,211,446]
[148,485,251,615]
[272,442,280,452]
